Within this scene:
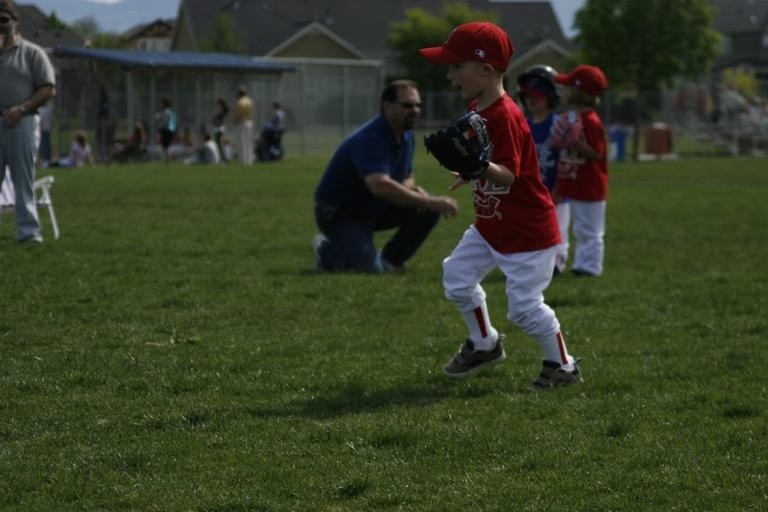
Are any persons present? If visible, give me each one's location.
[517,64,561,194]
[1,1,58,247]
[550,64,609,275]
[312,80,458,277]
[36,83,286,171]
[417,21,583,393]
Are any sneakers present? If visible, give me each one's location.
[545,368,550,372]
[531,356,584,390]
[441,333,508,379]
[313,232,409,274]
[18,231,43,244]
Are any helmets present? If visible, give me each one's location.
[515,63,561,105]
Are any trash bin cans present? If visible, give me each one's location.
[608,129,627,161]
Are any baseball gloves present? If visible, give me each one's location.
[424,111,491,178]
[548,113,584,151]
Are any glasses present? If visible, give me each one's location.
[0,16,10,25]
[392,99,425,110]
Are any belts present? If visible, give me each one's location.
[0,110,40,116]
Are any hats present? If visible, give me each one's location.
[417,22,514,72]
[552,62,610,98]
[0,1,19,20]
[517,78,552,101]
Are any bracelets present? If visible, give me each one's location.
[20,103,28,114]
